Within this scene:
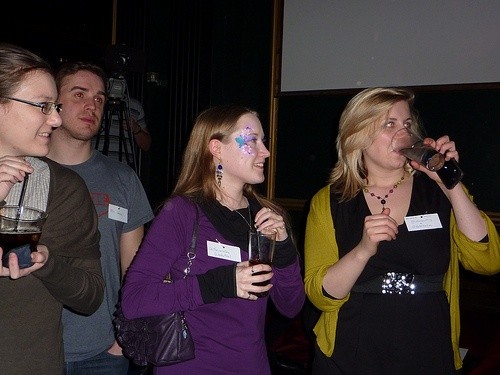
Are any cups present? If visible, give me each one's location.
[247,229,278,298]
[0,206,49,269]
[390,127,445,172]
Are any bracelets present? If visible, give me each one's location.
[133,127,141,135]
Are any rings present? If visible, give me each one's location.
[267,208,272,212]
[281,221,285,229]
[247,293,250,299]
[251,266,254,273]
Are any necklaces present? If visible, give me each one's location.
[215,196,253,231]
[211,176,243,207]
[363,171,409,211]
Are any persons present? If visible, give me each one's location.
[91,96,153,166]
[45,59,155,375]
[121,108,305,375]
[303,87,500,375]
[0,41,106,375]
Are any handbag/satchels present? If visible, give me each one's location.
[113,197,199,365]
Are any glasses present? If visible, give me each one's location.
[0,94,62,116]
